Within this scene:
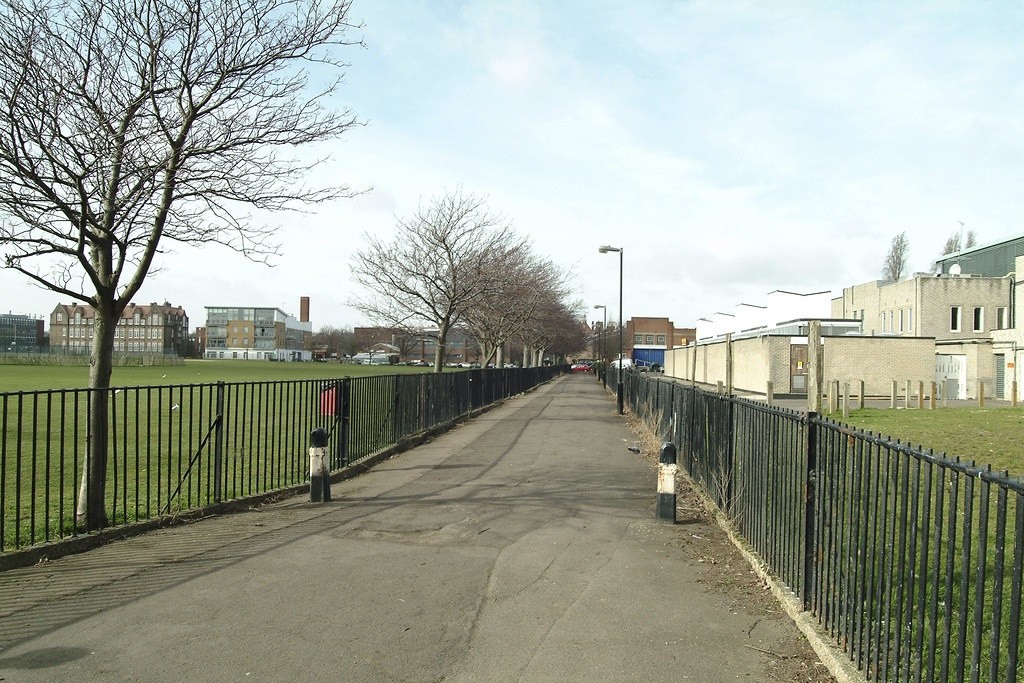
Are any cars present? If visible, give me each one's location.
[392,359,519,369]
[570,363,592,371]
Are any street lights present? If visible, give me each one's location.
[597,245,625,414]
[594,305,607,388]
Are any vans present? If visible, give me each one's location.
[610,359,634,373]
